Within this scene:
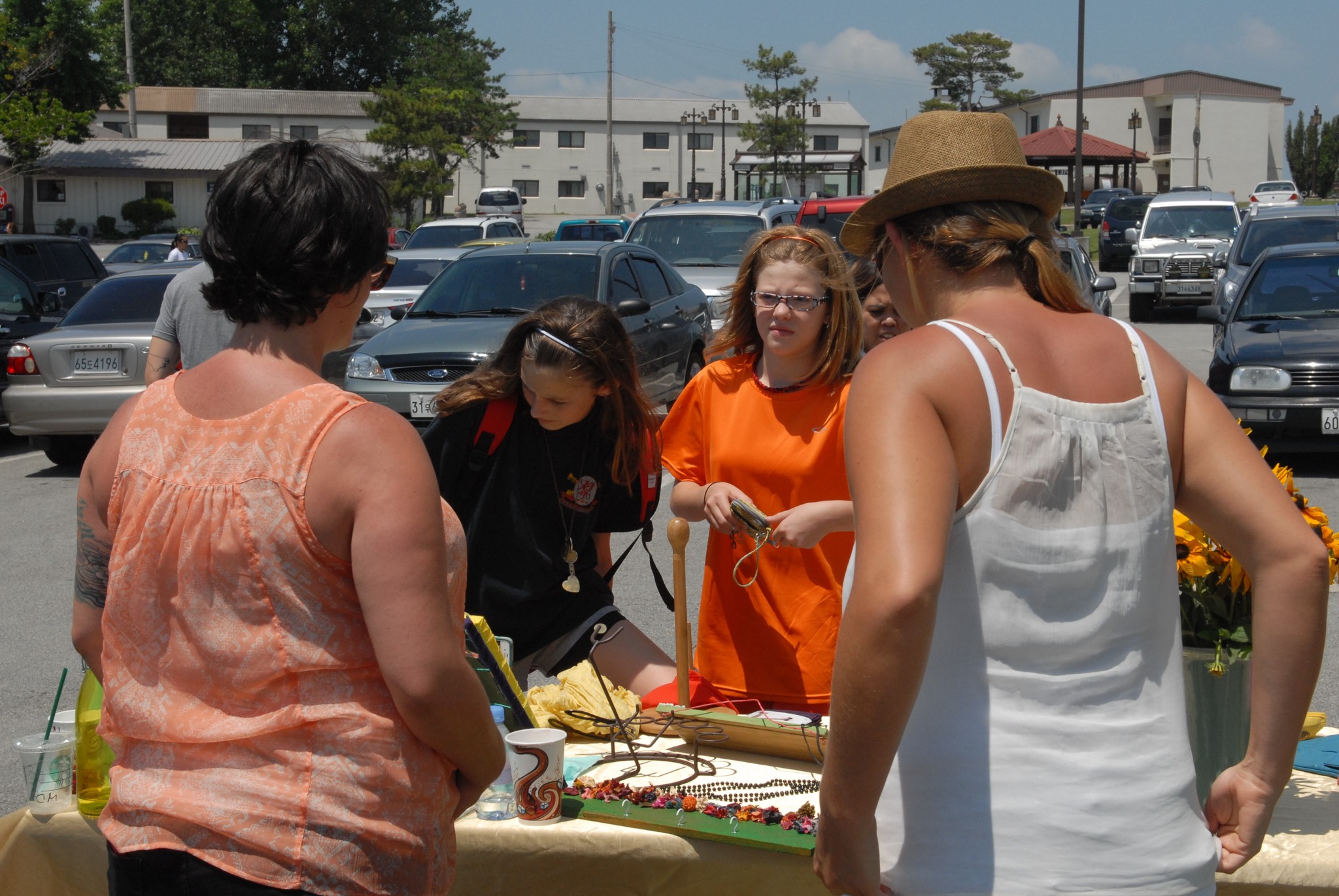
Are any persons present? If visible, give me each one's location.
[6,214,18,235]
[71,138,505,896]
[656,225,862,716]
[168,234,189,261]
[145,262,239,388]
[420,298,678,701]
[827,257,911,355]
[812,111,1329,896]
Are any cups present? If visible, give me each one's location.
[48,709,104,794]
[13,732,76,815]
[505,728,567,826]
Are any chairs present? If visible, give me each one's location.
[1263,286,1315,312]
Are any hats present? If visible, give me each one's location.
[839,110,1063,257]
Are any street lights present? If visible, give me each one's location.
[680,109,708,198]
[707,98,739,201]
[1127,108,1142,195]
[787,92,822,197]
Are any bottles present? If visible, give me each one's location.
[75,657,115,819]
[475,705,517,821]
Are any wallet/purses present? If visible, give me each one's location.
[730,499,779,587]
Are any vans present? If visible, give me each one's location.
[473,185,528,233]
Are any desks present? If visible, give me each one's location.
[0,735,829,896]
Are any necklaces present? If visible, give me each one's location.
[544,429,590,593]
[751,351,827,394]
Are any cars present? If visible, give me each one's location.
[1205,241,1339,461]
[551,218,639,242]
[0,257,80,434]
[98,213,526,329]
[0,269,390,467]
[1078,188,1135,229]
[1246,181,1308,208]
[1167,185,1212,193]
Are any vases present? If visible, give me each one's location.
[1184,646,1250,806]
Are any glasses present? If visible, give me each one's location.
[366,251,398,291]
[181,240,188,243]
[750,290,831,312]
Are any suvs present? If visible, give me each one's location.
[1209,200,1339,347]
[1124,190,1249,321]
[794,193,879,257]
[0,233,116,312]
[614,196,806,361]
[1092,194,1158,270]
[343,238,713,439]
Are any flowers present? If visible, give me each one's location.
[1172,419,1338,675]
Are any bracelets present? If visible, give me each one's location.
[703,481,720,505]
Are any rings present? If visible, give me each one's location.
[733,524,735,531]
